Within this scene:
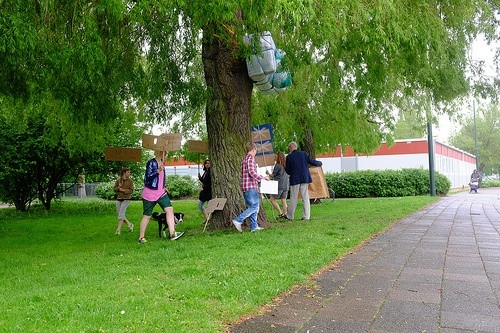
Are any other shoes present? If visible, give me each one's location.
[284,215,293,221]
[300,217,304,220]
[202,218,212,224]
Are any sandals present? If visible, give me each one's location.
[276,213,284,220]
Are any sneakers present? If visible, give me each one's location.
[251,224,265,233]
[169,231,185,240]
[115,229,121,236]
[232,220,243,233]
[138,237,148,245]
[127,224,134,233]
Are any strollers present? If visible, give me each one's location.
[469,179,479,193]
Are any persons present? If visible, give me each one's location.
[259,141,290,220]
[198,159,212,224]
[138,148,184,244]
[113,167,134,235]
[471,169,480,193]
[231,143,264,233]
[283,141,323,222]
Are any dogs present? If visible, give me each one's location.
[151,211,184,237]
